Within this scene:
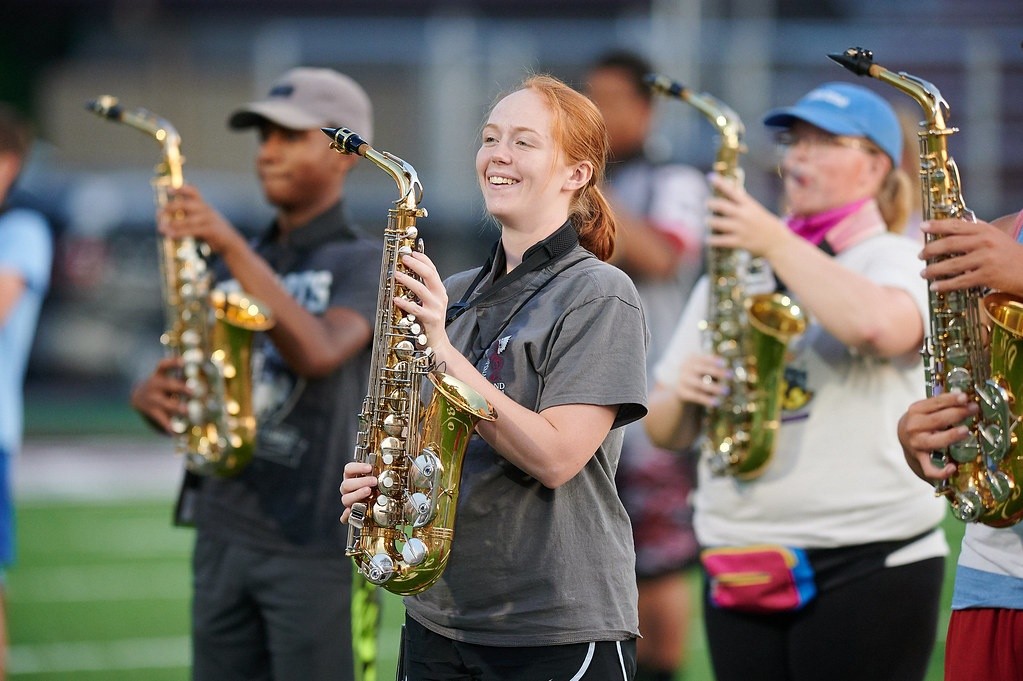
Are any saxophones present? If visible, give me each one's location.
[322,124,498,593]
[86,92,280,473]
[837,44,1022,524]
[649,77,808,482]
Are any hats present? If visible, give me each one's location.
[762,81,901,169]
[229,67,373,147]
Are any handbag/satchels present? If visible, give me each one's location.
[699,527,950,610]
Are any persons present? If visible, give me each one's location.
[582,56,708,681]
[0,126,52,681]
[646,81,950,681]
[339,76,651,681]
[132,67,384,681]
[898,209,1023,681]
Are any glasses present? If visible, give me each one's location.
[771,129,879,162]
